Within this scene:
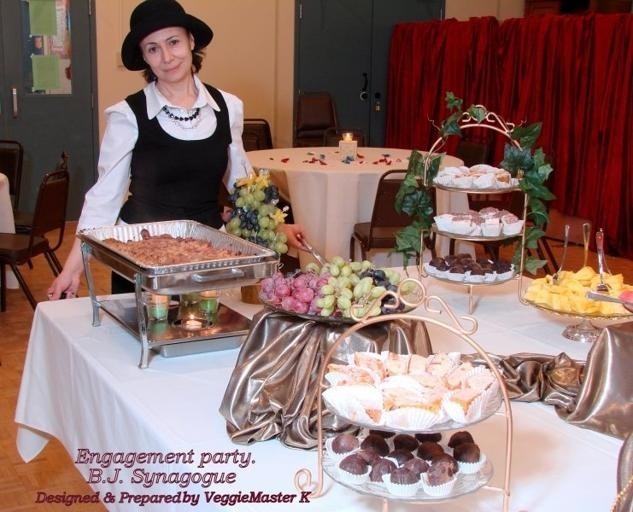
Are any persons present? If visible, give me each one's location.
[48,0,313,301]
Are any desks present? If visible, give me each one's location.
[34,263,633,511]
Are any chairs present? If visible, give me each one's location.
[487,163,561,277]
[241,118,274,153]
[292,89,340,148]
[0,139,24,220]
[0,170,79,314]
[322,126,368,150]
[348,168,439,269]
[449,192,537,271]
[453,142,490,171]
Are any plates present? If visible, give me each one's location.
[321,453,493,502]
[424,256,515,287]
[430,214,520,244]
[322,392,502,432]
[255,294,419,325]
[433,169,520,195]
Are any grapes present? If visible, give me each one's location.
[259,255,416,319]
[226,180,289,253]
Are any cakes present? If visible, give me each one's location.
[448,207,518,224]
[429,253,512,274]
[327,349,496,486]
[496,172,510,184]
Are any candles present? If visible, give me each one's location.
[184,313,202,330]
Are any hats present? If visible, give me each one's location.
[120,0,214,72]
